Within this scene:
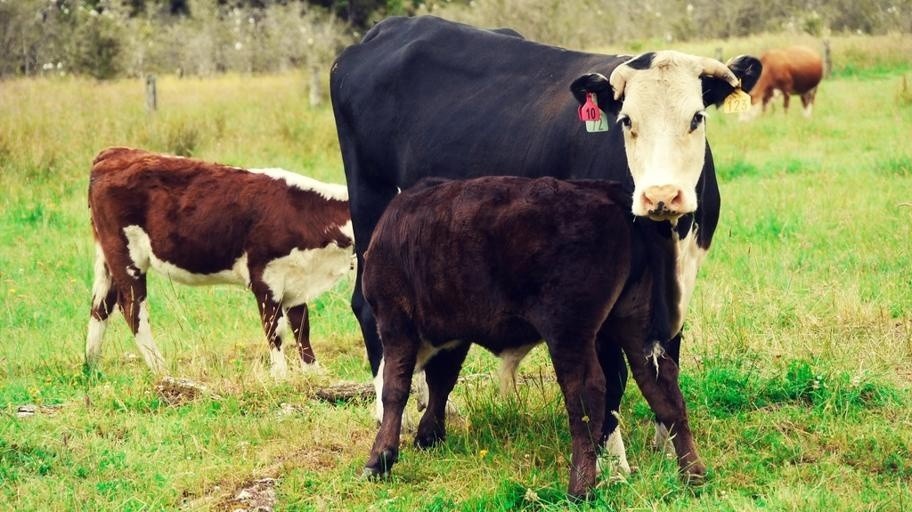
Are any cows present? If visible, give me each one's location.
[362,174,708,505]
[724,55,762,94]
[329,14,763,474]
[749,46,823,117]
[85,146,357,381]
